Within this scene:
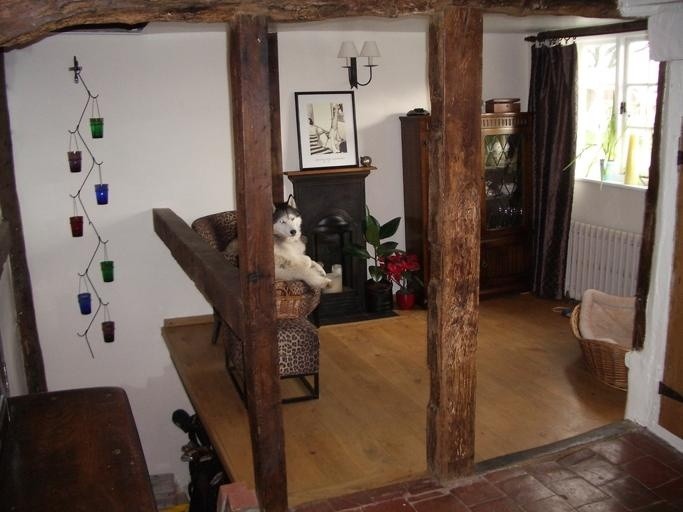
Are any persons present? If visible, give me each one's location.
[172,409,211,480]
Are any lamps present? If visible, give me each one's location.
[336,41,381,89]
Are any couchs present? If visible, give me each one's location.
[194,200,324,406]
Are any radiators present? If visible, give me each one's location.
[564,220,642,301]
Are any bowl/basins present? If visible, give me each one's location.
[497,183,518,194]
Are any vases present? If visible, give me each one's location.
[395,290,416,311]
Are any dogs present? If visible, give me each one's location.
[223,191,333,302]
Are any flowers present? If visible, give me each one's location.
[380,252,420,291]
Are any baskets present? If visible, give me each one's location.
[570,302,632,392]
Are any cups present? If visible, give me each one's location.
[100,320,114,343]
[73,217,83,238]
[100,259,114,283]
[95,186,110,205]
[79,295,90,315]
[91,118,105,138]
[69,150,81,172]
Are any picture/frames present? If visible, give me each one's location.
[294,91,360,171]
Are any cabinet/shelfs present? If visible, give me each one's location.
[397,110,536,304]
[279,164,374,307]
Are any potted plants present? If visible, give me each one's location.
[349,212,401,311]
[561,89,632,189]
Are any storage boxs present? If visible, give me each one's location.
[486,97,521,113]
